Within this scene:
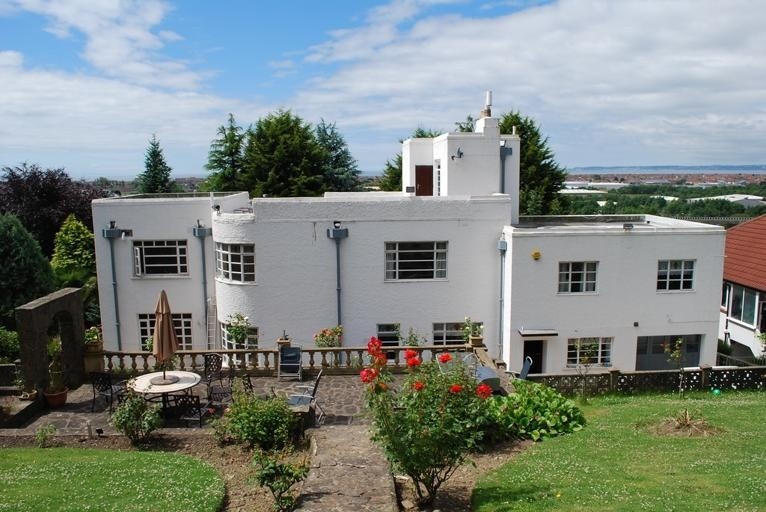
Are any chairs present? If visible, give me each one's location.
[286,369,328,428]
[90,371,124,414]
[502,356,533,391]
[276,344,303,382]
[173,353,234,429]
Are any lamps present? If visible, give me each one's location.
[451,147,463,161]
[212,204,222,216]
[623,223,633,233]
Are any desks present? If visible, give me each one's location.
[127,370,202,410]
[435,351,484,393]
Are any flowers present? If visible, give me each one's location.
[465,315,485,337]
[84,327,103,343]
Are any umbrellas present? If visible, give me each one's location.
[153,289,179,380]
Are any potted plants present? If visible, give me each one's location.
[277,330,292,351]
[42,337,70,409]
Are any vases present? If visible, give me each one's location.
[468,335,484,346]
[85,340,103,352]
